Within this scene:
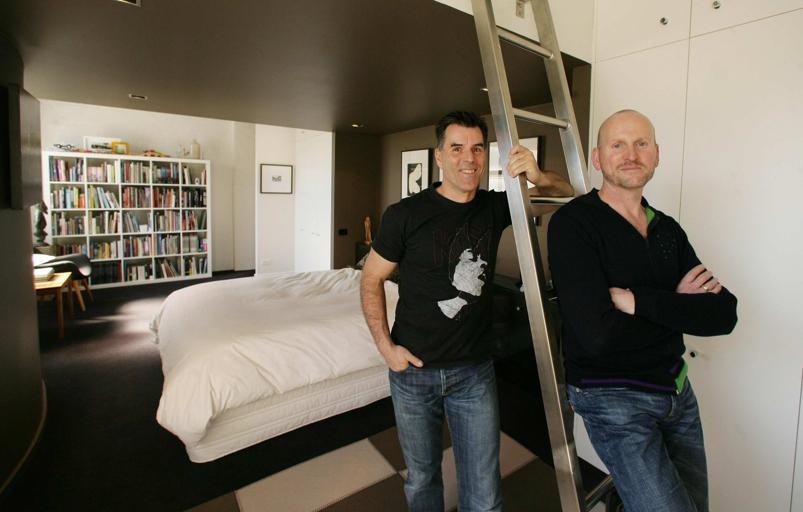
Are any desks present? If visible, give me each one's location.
[35,272,77,336]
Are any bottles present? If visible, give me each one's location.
[189,140,201,159]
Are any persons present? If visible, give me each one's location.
[363,216,372,241]
[546,108,739,512]
[359,109,574,511]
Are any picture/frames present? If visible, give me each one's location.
[487,137,542,224]
[112,142,129,153]
[260,164,292,195]
[398,147,430,199]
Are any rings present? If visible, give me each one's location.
[703,284,710,291]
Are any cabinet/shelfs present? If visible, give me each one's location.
[36,149,214,296]
[355,243,371,266]
[492,275,576,468]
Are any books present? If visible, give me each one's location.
[48,156,208,285]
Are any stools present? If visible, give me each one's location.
[34,253,93,314]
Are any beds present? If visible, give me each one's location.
[162,201,567,467]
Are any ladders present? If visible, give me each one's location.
[471,0,619,512]
